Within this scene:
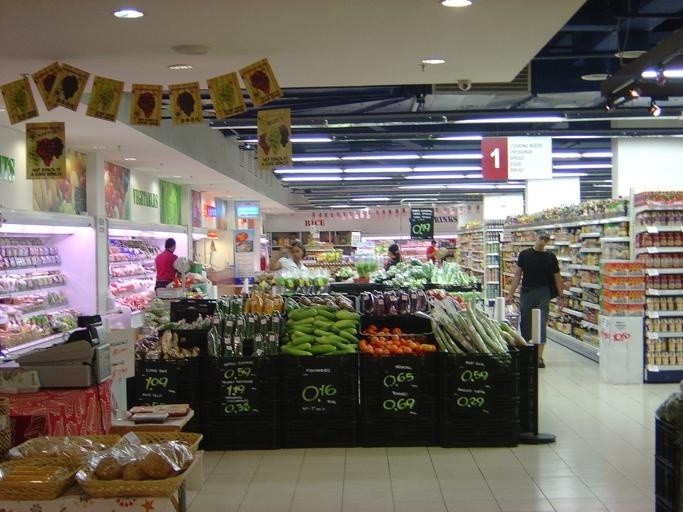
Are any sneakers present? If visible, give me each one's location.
[537,357,545,368]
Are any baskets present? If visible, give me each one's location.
[7,434,119,459]
[112,430,203,459]
[0,460,85,501]
[74,455,198,497]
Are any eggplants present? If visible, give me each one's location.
[361,287,428,315]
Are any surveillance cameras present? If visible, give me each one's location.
[459,79,471,91]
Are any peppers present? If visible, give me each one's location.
[206,281,284,358]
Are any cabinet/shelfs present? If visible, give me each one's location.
[0,208,98,365]
[106,218,188,329]
[455,192,683,386]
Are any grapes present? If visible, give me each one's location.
[11,69,290,167]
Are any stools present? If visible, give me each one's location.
[111,408,195,433]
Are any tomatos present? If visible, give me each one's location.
[358,323,437,358]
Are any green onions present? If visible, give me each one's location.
[431,290,512,366]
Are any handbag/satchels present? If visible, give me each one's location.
[546,270,559,299]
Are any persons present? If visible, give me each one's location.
[506,231,562,369]
[426,240,437,263]
[384,244,406,271]
[154,237,178,292]
[269,239,307,272]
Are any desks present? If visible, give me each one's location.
[0,378,113,447]
[1,450,204,512]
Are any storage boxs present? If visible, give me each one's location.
[192,290,548,445]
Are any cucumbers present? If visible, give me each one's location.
[281,304,361,357]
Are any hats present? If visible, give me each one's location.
[536,230,550,238]
